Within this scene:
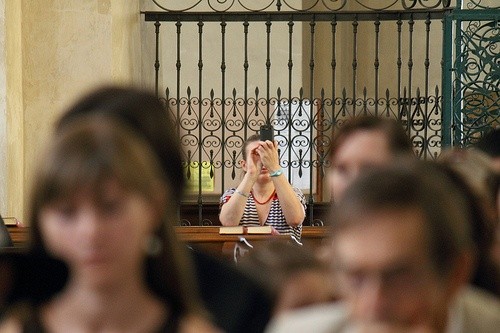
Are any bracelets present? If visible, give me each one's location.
[234,188,250,198]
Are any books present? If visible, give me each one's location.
[246,226,280,235]
[218,225,245,236]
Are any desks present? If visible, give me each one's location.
[8,226,328,254]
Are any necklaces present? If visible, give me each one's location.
[250,189,276,205]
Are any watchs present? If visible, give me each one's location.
[269,168,284,177]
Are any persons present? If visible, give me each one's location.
[218,133,307,246]
[1,84,500,333]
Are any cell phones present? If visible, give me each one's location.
[260,125,274,144]
